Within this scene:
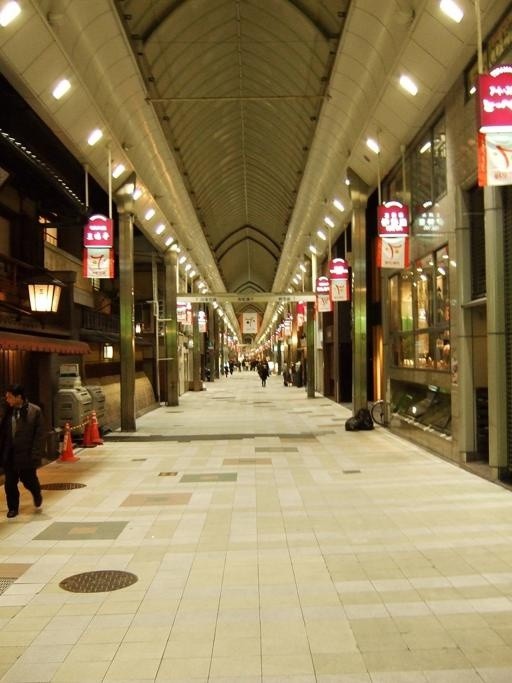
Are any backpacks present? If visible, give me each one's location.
[345,408,375,431]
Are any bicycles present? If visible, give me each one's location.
[371,389,425,427]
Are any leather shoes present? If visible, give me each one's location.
[34,494,43,507]
[6,509,18,517]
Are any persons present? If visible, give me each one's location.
[0,382,46,517]
[223,356,271,388]
[283,360,290,387]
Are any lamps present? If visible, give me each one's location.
[0,266,66,323]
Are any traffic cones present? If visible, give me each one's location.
[79,409,104,448]
[58,422,80,463]
